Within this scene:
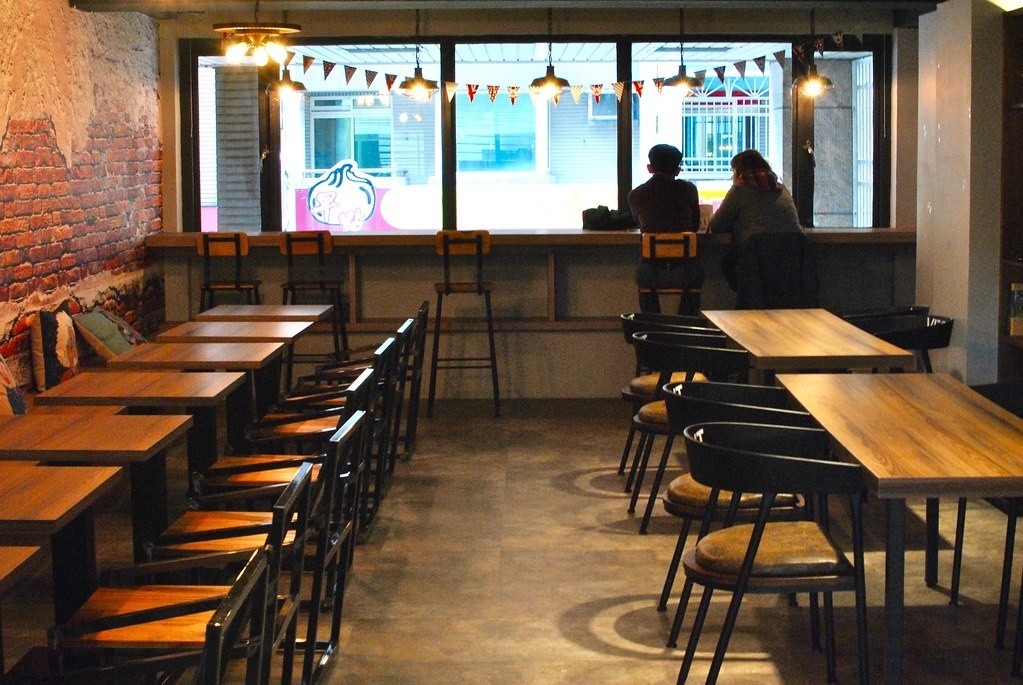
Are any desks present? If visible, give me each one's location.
[775,371,1023,685]
[105,343,285,497]
[0,465,158,685]
[156,321,315,447]
[0,413,222,588]
[702,308,914,389]
[195,305,335,420]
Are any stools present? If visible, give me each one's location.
[634,231,702,377]
[279,229,349,393]
[196,232,260,315]
[425,229,502,417]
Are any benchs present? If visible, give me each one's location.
[0,320,188,593]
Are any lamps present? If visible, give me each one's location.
[212,0,302,67]
[529,7,570,97]
[266,10,307,101]
[661,8,702,95]
[399,8,439,101]
[792,10,834,96]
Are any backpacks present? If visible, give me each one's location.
[582,205,636,231]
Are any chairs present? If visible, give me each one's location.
[619,311,868,685]
[47,300,433,685]
[949,496,1023,678]
[852,313,954,374]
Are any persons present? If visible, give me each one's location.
[628,144,705,315]
[708,150,820,308]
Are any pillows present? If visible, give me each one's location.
[76,309,149,361]
[29,297,81,391]
[0,354,28,416]
